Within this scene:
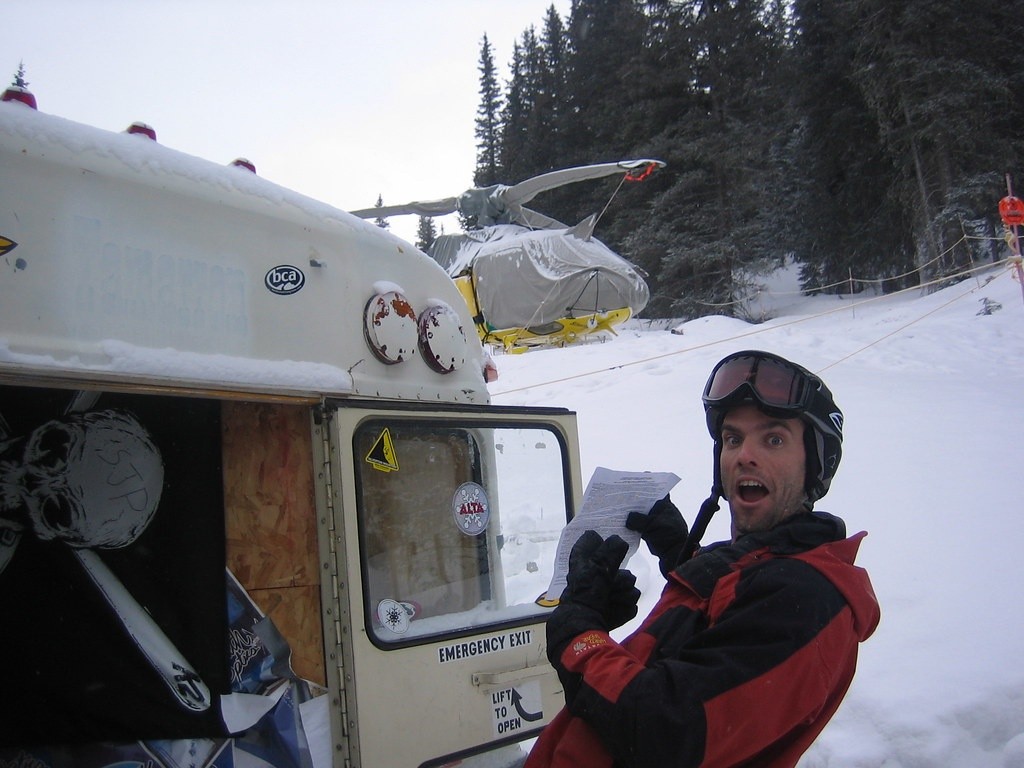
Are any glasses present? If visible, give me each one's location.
[701,350,811,418]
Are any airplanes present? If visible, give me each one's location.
[339,158,669,356]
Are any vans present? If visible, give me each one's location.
[0,89,585,768]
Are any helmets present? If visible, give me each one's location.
[706,361,843,501]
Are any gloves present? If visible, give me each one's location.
[625,471,698,573]
[546,530,641,663]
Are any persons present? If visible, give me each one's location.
[524,351,881,768]
